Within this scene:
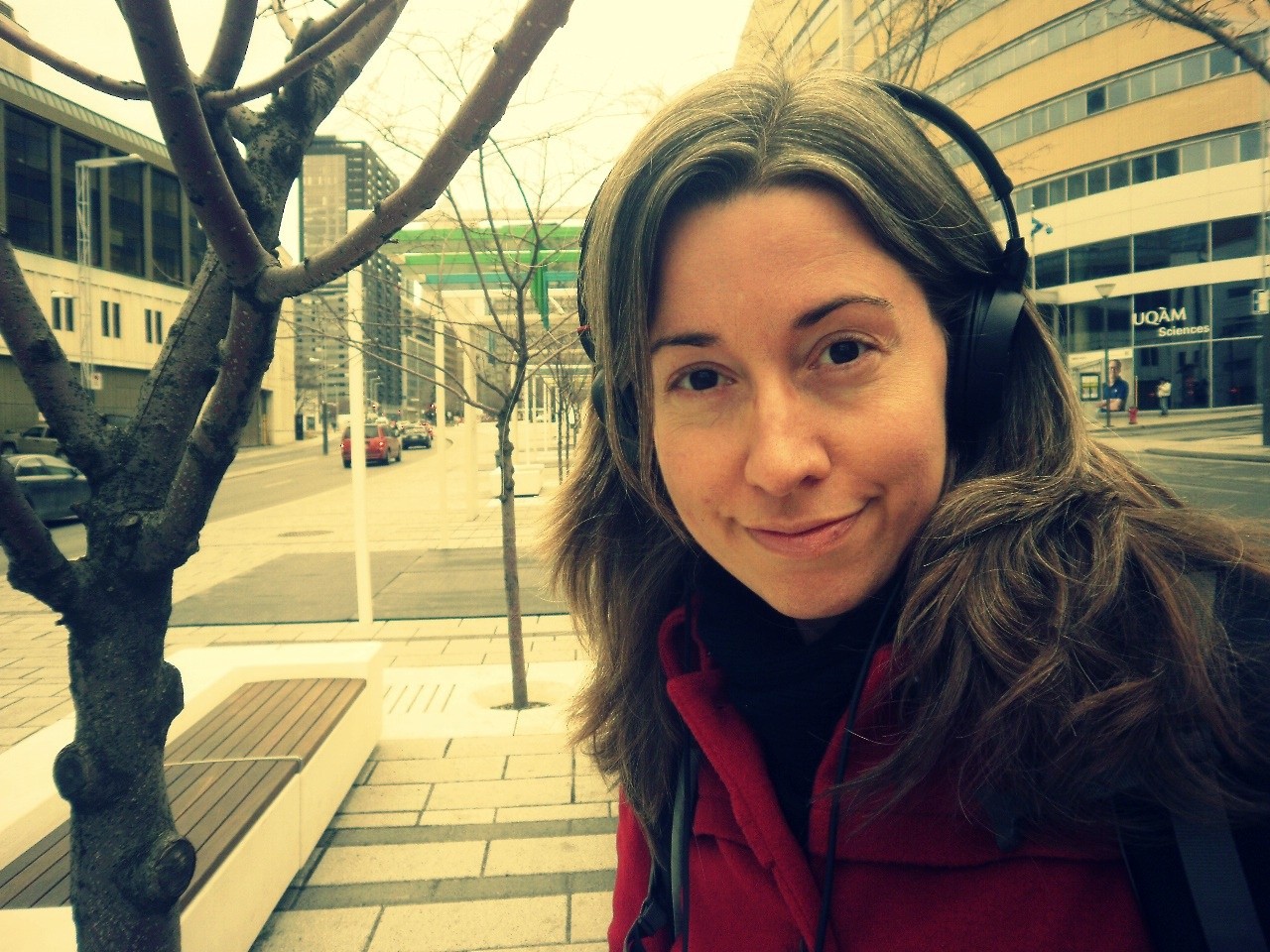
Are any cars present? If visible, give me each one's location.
[0,420,74,465]
[1,454,92,522]
[340,423,402,468]
[395,421,433,450]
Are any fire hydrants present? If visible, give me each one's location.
[1128,406,1138,425]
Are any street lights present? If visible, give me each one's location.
[75,154,147,404]
[1094,282,1115,428]
[1029,220,1053,311]
[365,370,383,414]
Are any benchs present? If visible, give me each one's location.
[0,680,377,952]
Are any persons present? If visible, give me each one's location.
[1157,377,1172,415]
[1098,359,1129,413]
[531,70,1270,952]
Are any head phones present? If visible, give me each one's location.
[575,81,1030,440]
